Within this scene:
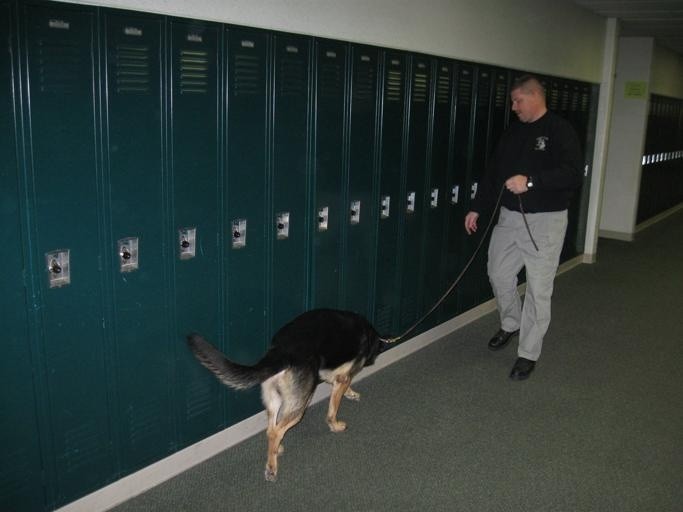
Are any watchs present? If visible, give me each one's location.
[526,176,534,192]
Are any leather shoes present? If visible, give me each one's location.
[487,328,520,351]
[509,357,536,381]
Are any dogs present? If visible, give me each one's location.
[184,306,392,483]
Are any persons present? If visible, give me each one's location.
[462,74,585,383]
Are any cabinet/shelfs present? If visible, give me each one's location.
[632,92,683,234]
[1,0,600,512]
